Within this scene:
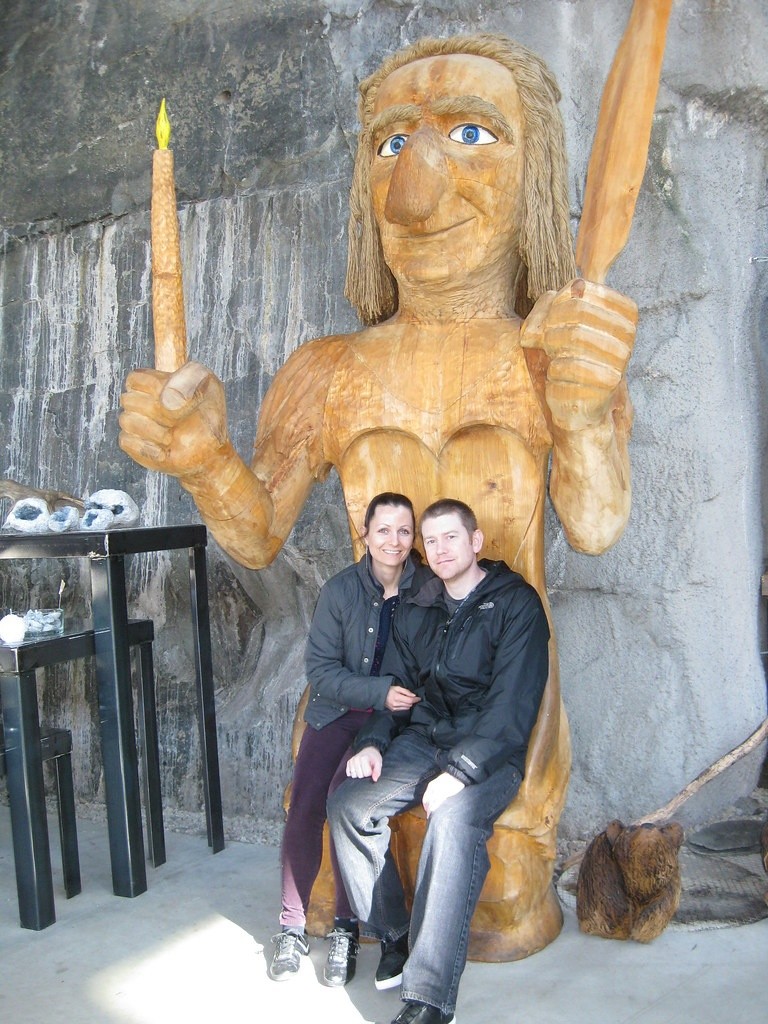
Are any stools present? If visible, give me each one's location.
[0,727,84,899]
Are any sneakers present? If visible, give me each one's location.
[268,928,311,981]
[323,928,356,987]
[374,931,408,990]
[391,1000,457,1024]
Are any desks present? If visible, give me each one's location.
[0,618,167,932]
[0,524,225,899]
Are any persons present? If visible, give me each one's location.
[119,37,639,968]
[324,498,553,1024]
[268,492,439,990]
